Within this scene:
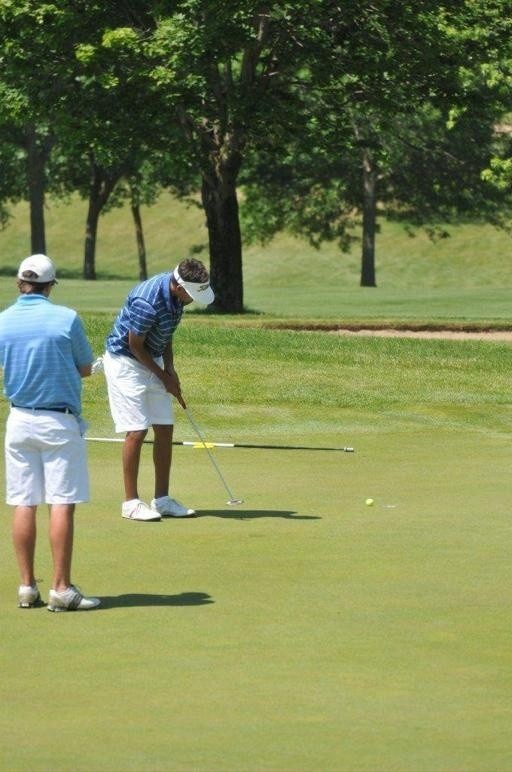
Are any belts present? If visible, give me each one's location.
[11,402,73,414]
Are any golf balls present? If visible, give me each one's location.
[176,394,244,507]
[366,498,374,505]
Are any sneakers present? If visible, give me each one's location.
[48,585,101,611]
[122,498,161,521]
[152,496,195,517]
[18,585,47,607]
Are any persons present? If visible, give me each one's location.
[0,254,101,612]
[104,259,215,521]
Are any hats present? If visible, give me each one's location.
[174,265,215,304]
[18,253,60,287]
